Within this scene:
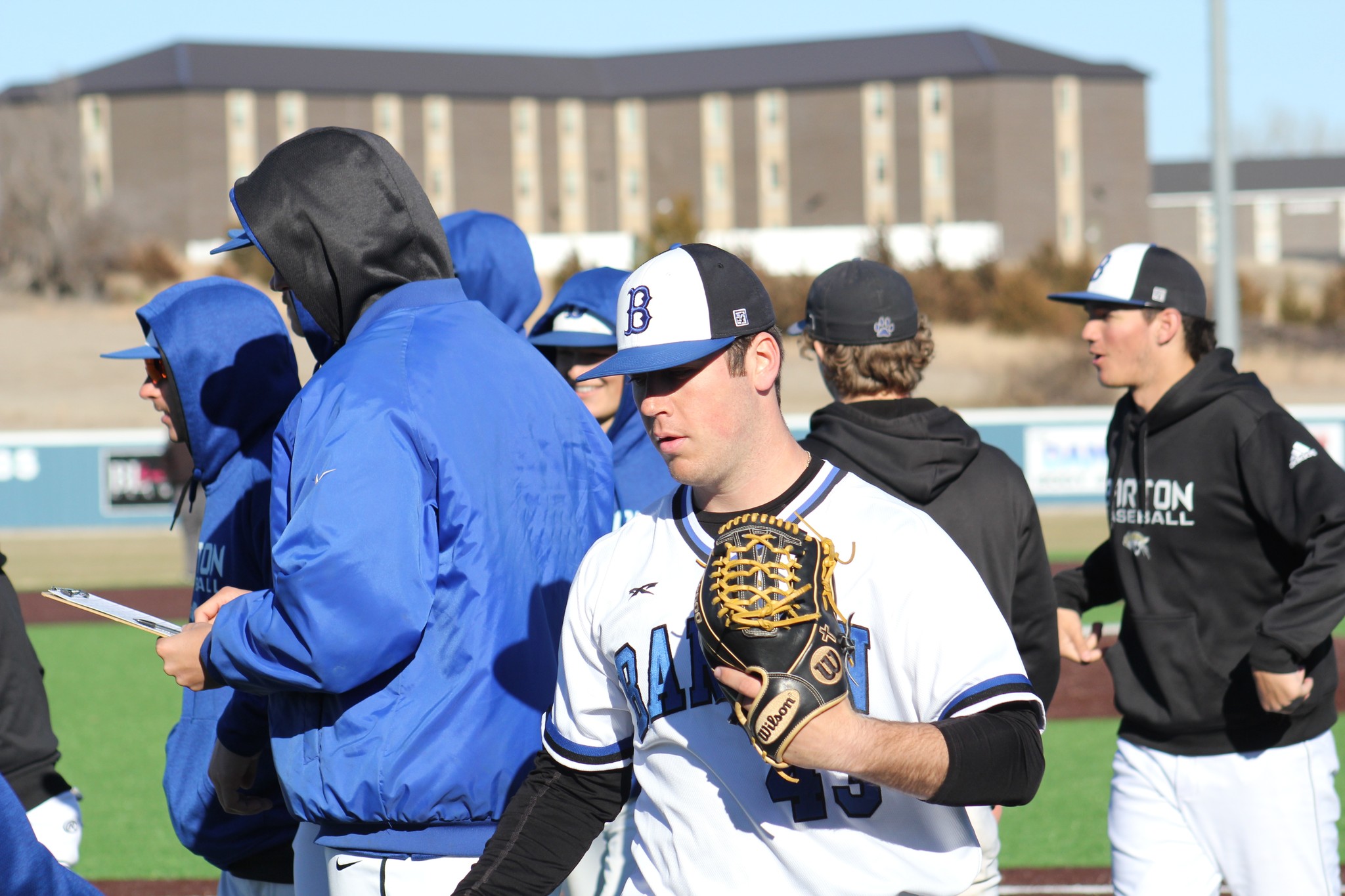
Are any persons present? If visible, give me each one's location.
[0,552,82,871]
[0,775,105,896]
[1049,244,1345,896]
[101,278,300,896]
[153,126,622,896]
[451,242,1044,894]
[528,267,682,533]
[439,209,541,341]
[791,259,1061,821]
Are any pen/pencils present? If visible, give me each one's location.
[133,618,182,634]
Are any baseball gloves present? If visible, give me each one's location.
[690,504,861,787]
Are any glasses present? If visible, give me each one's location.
[143,360,168,388]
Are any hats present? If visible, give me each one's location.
[227,229,250,239]
[210,232,251,255]
[100,328,161,360]
[787,257,918,345]
[528,307,617,347]
[574,242,776,382]
[1046,242,1207,320]
